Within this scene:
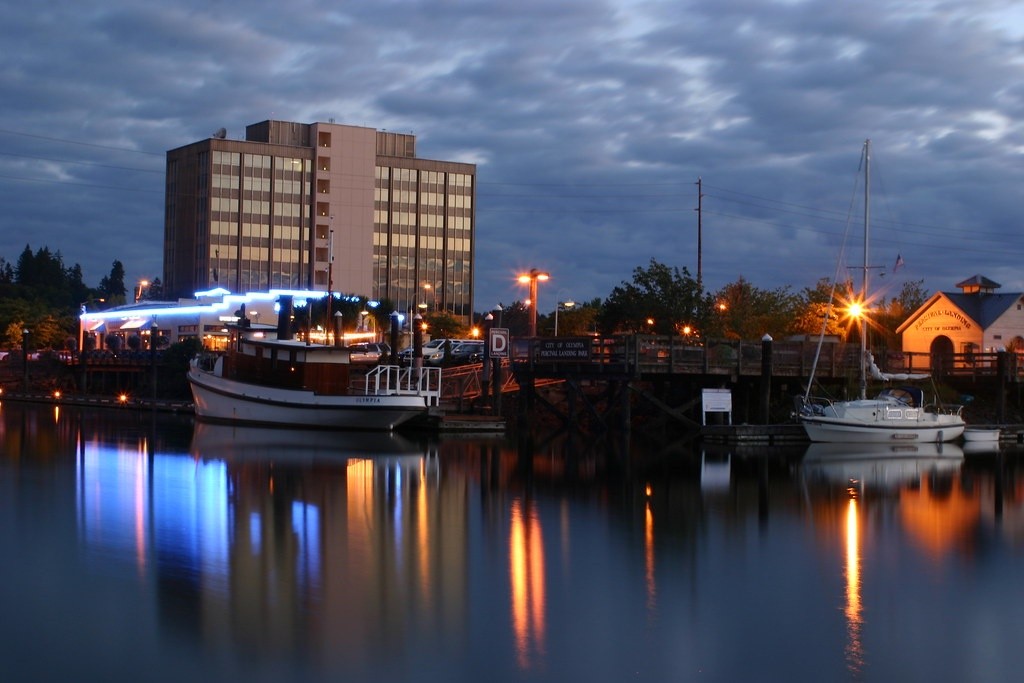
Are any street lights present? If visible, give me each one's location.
[517,271,549,337]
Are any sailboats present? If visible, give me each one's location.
[787,139,968,443]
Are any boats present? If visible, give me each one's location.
[963,441,1000,453]
[963,428,1000,442]
[185,225,443,434]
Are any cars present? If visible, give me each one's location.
[397,339,509,371]
